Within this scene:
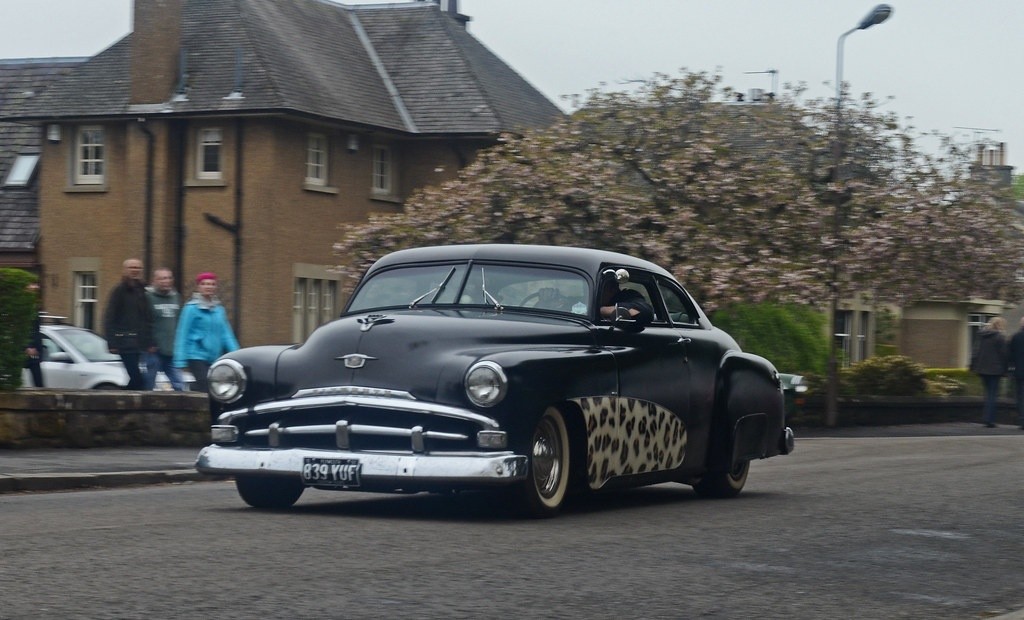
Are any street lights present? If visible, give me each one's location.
[827,4,893,434]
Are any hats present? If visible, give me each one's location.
[196,273,218,283]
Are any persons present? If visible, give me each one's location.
[144,269,184,391]
[104,259,157,390]
[173,272,240,393]
[970,316,1024,430]
[538,272,655,324]
[23,284,45,387]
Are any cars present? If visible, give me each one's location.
[195,244,797,522]
[777,373,808,393]
[22,324,195,394]
